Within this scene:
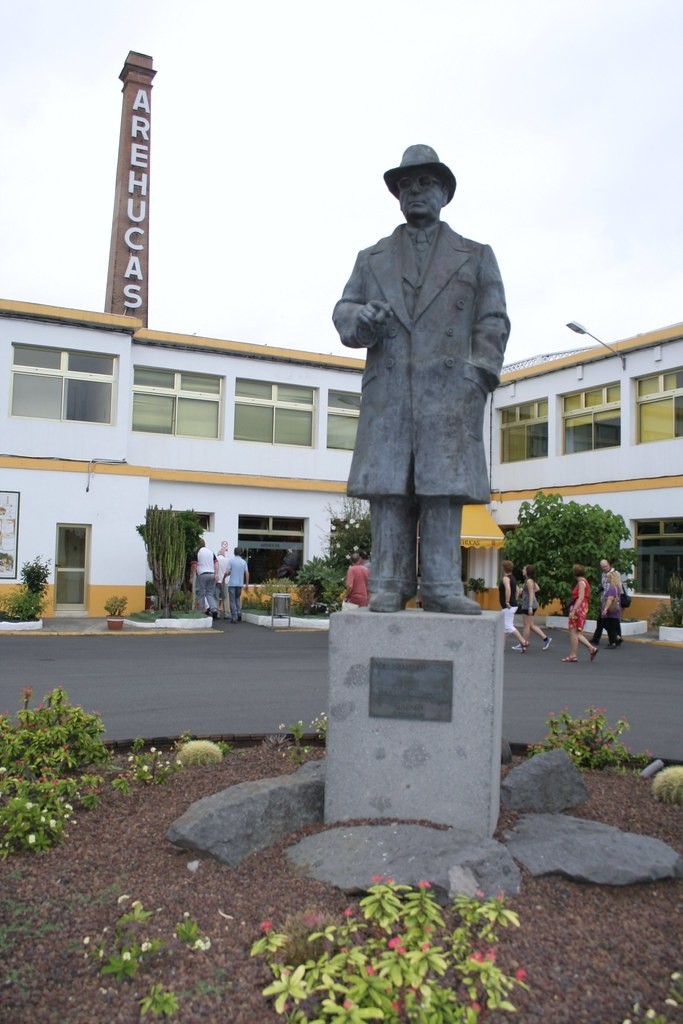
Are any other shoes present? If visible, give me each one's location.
[604,636,624,650]
[230,619,238,624]
[211,609,218,622]
[587,638,600,645]
[237,613,242,622]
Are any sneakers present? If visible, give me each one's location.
[542,637,551,650]
[512,644,522,650]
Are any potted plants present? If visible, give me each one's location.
[104,596,129,630]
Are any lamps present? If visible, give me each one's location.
[566,321,626,370]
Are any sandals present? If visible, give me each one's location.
[520,640,531,654]
[562,655,578,662]
[590,647,599,662]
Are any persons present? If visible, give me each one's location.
[189,538,250,625]
[332,144,512,616]
[500,559,623,664]
[340,549,372,612]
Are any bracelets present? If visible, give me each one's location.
[506,602,510,604]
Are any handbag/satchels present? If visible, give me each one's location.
[620,593,631,609]
[602,584,619,615]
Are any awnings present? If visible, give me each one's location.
[461,504,505,549]
